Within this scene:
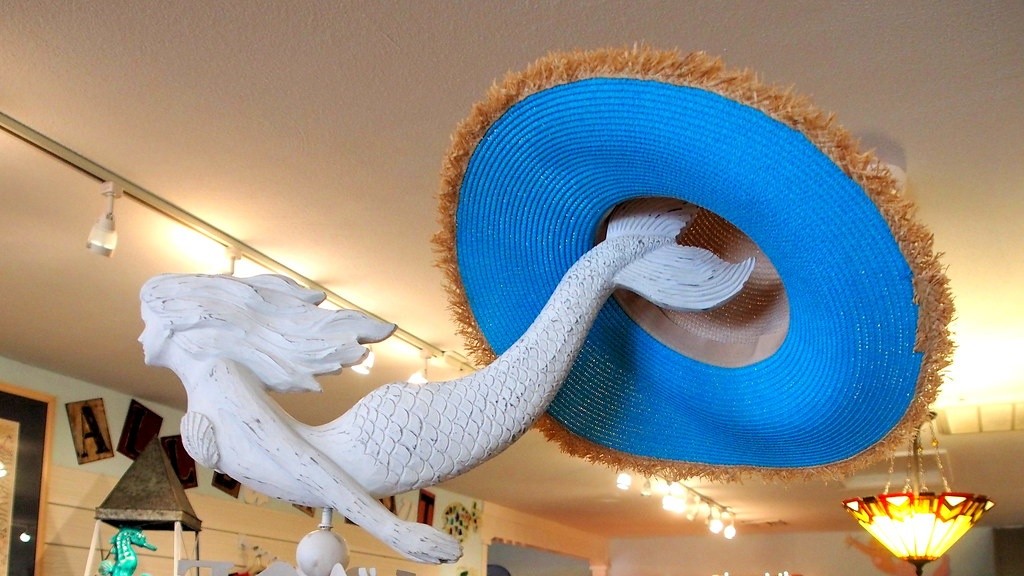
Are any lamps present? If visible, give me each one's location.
[408,349,431,385]
[842,410,996,576]
[351,344,375,376]
[616,467,736,539]
[85,181,124,257]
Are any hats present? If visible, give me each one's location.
[435,50,953,483]
